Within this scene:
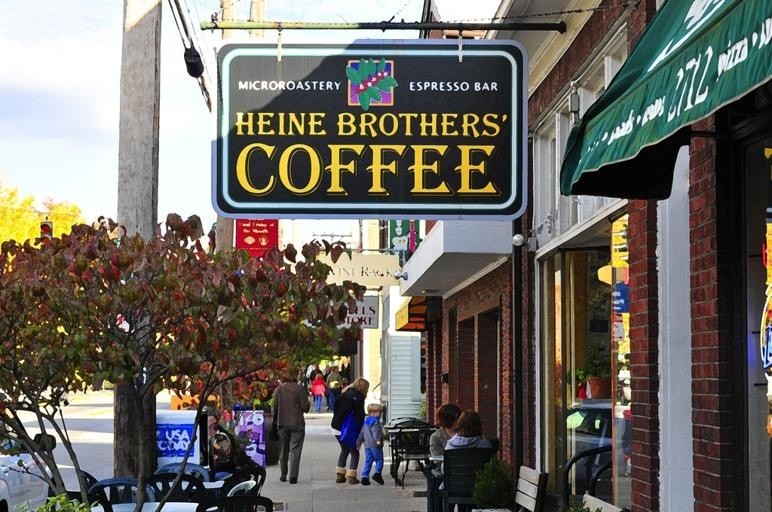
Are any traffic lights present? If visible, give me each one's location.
[40,221,52,246]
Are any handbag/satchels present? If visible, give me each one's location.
[340,415,357,450]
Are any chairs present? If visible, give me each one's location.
[45,458,274,512]
[388,415,494,512]
[471,465,548,512]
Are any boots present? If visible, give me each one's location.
[347,469,360,484]
[335,466,347,483]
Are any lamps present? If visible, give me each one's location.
[512,233,537,254]
[393,270,408,281]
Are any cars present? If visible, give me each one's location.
[0,393,58,512]
[561,399,613,490]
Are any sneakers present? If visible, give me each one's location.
[361,478,371,486]
[372,473,384,485]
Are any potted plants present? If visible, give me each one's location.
[574,367,588,401]
[583,335,611,400]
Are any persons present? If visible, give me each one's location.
[308,364,351,413]
[330,377,369,484]
[271,367,311,484]
[436,408,492,512]
[356,403,385,485]
[429,404,463,512]
